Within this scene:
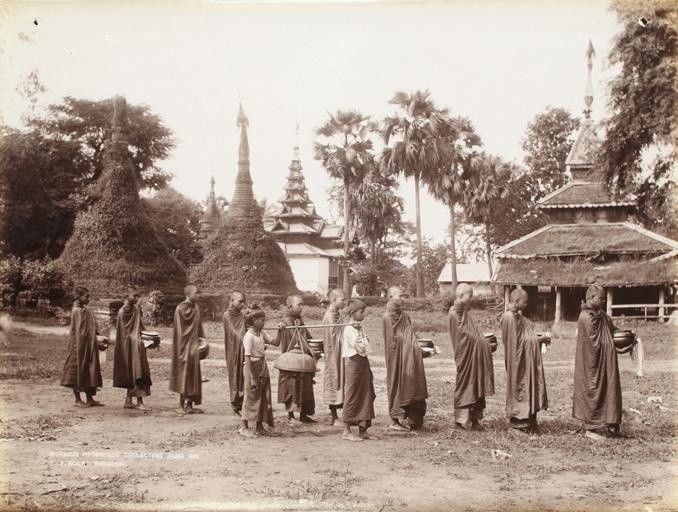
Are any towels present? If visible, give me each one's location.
[631,337,645,376]
[540,332,552,354]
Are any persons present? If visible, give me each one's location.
[278,295,319,424]
[322,288,348,427]
[448,283,498,431]
[381,286,437,430]
[341,300,376,441]
[60,286,106,408]
[112,288,160,411]
[222,291,248,417]
[169,284,205,415]
[500,288,550,429]
[572,285,637,441]
[239,310,277,439]
